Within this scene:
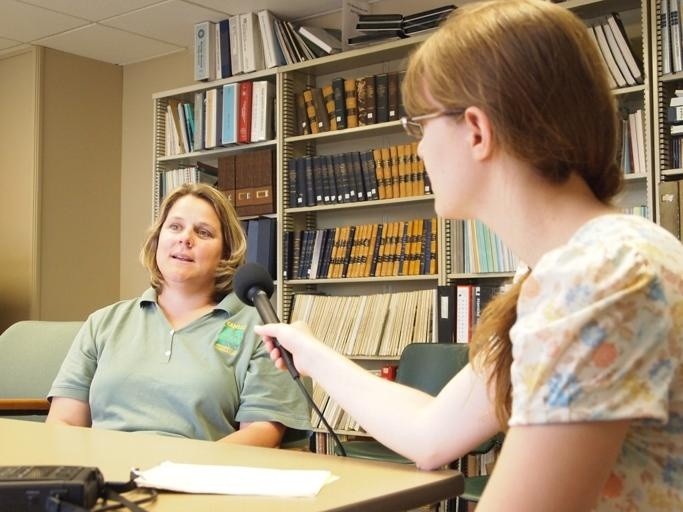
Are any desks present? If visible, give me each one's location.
[0,417,465,512]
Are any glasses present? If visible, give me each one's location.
[399,109,468,139]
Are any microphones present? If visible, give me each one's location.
[234,263,300,382]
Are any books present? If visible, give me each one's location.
[194,8,342,82]
[289,71,438,454]
[587,0,683,244]
[348,4,457,49]
[480,450,496,477]
[457,221,518,345]
[164,80,277,280]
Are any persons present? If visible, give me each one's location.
[42,181,315,449]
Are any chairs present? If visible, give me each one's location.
[332,341,498,512]
[1,320,88,422]
